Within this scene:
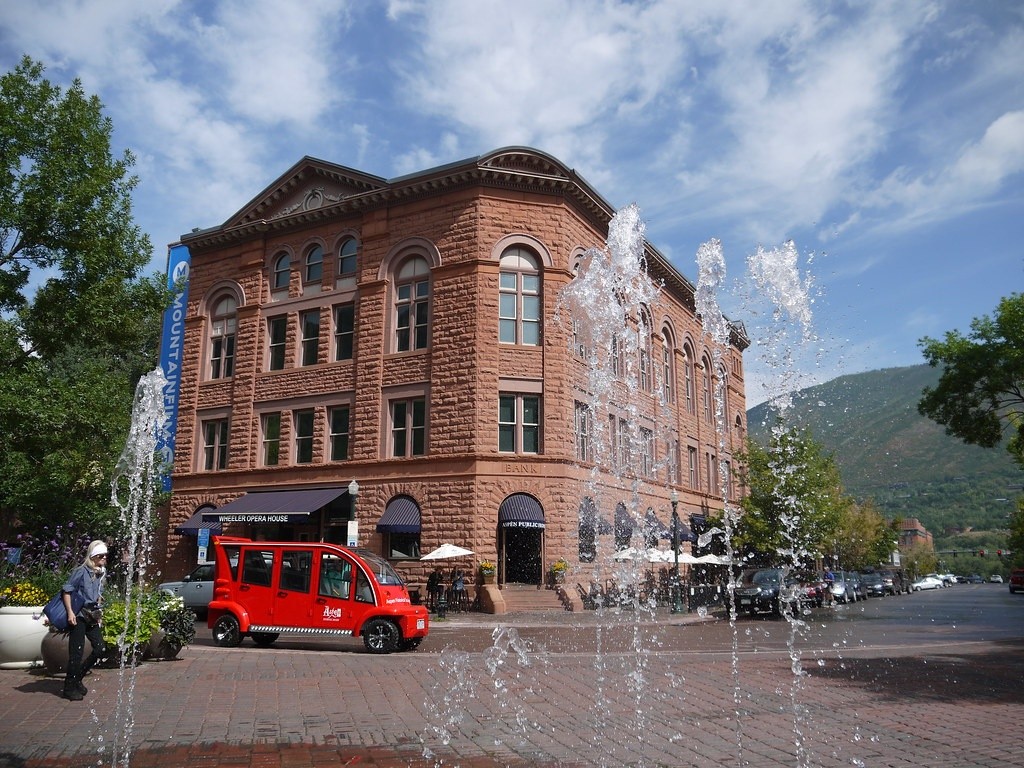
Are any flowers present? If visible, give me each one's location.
[0,582,50,607]
[479,561,496,574]
[550,558,570,574]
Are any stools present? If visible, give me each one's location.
[427,590,443,611]
[444,589,471,616]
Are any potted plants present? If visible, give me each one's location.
[144,591,197,661]
[96,599,160,666]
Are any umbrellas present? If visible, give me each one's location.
[605,546,747,585]
[391,550,409,558]
[419,543,476,580]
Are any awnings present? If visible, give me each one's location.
[201,488,349,523]
[578,497,710,542]
[174,507,222,536]
[375,495,421,533]
[499,493,546,528]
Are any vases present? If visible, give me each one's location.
[552,571,566,584]
[0,606,50,669]
[41,627,94,676]
[482,575,495,585]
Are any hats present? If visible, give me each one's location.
[824,566,829,570]
[90,544,108,558]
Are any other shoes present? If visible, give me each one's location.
[75,681,87,694]
[63,687,82,700]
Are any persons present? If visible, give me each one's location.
[63,540,107,701]
[426,565,464,602]
[718,569,729,600]
[823,566,836,606]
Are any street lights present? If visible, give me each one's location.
[347,479,359,521]
[669,487,686,616]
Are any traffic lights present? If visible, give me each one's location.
[998,550,1002,556]
[980,550,984,557]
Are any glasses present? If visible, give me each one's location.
[95,554,107,559]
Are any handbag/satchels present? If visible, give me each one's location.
[80,606,102,626]
[43,572,85,631]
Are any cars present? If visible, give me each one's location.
[960,574,986,585]
[207,534,429,654]
[720,565,958,618]
[990,574,1004,584]
[157,558,295,622]
[1008,567,1024,593]
